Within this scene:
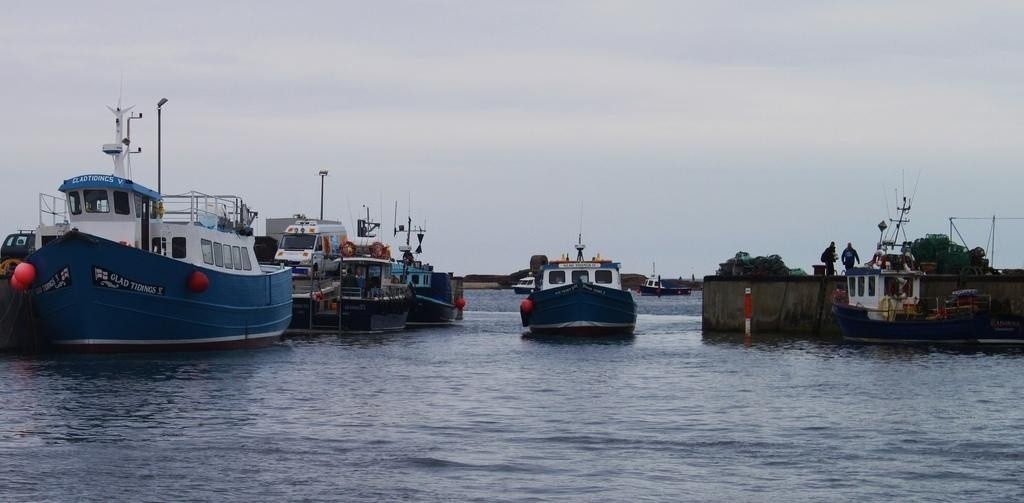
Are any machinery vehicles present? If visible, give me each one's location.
[342,218,391,258]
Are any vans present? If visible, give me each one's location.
[273,220,348,280]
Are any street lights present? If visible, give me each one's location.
[317,170,327,218]
[156,96,167,196]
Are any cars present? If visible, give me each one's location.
[252,235,278,265]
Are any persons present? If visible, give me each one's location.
[821,242,839,276]
[841,242,860,271]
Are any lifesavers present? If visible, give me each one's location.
[341,242,355,257]
[369,242,385,257]
[873,250,887,270]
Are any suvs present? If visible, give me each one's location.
[0,228,36,282]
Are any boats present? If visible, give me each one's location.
[389,189,456,326]
[519,200,638,340]
[511,271,535,294]
[10,70,292,357]
[831,166,1024,348]
[283,207,416,335]
[638,262,691,296]
